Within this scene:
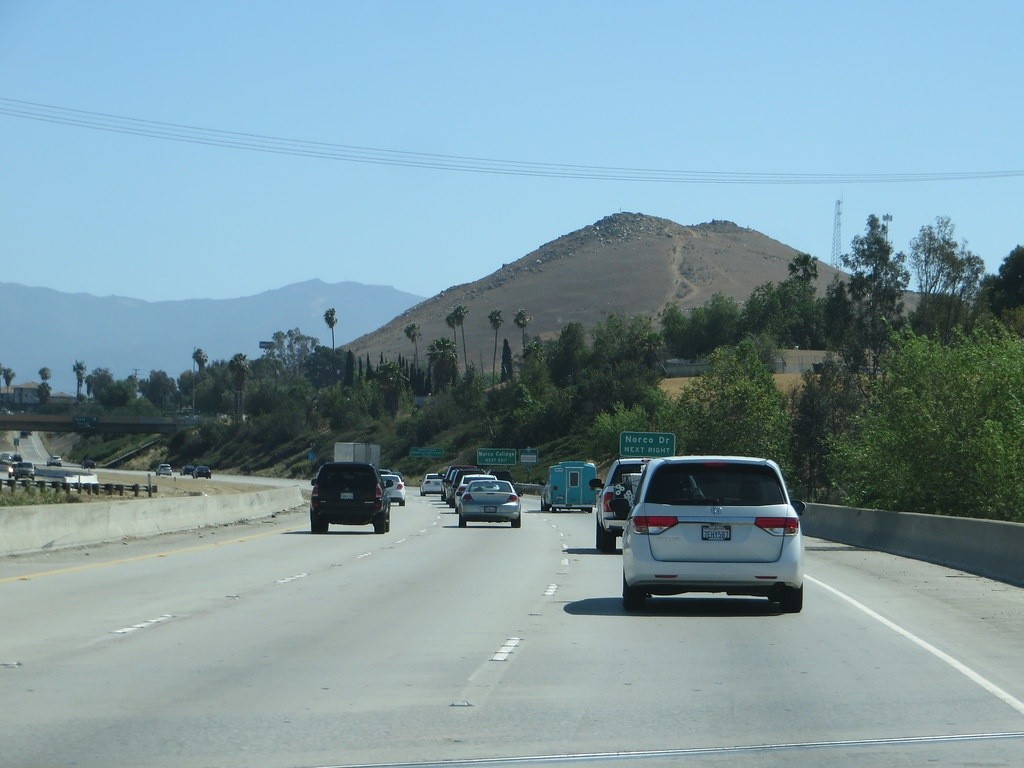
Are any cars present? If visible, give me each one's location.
[456,479,524,528]
[380,469,406,506]
[8,463,18,479]
[180,466,195,476]
[0,453,22,462]
[438,466,512,514]
[419,473,444,496]
[192,466,212,479]
[156,464,172,476]
[81,460,96,469]
[607,455,807,612]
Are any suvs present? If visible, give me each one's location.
[47,456,63,466]
[15,462,36,481]
[310,462,394,534]
[588,458,655,554]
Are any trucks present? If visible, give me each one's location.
[540,461,597,513]
